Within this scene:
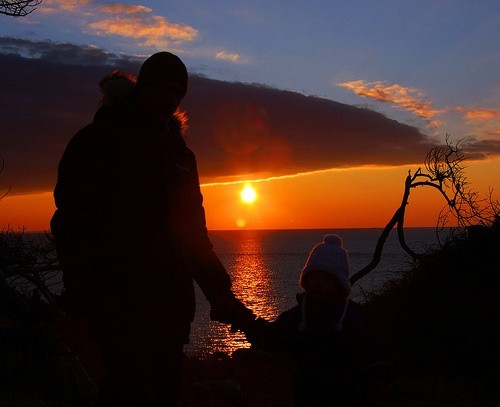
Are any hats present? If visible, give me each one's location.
[136,51,188,95]
[300,234,351,332]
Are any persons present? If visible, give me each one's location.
[227,236,375,407]
[50,52,235,407]
